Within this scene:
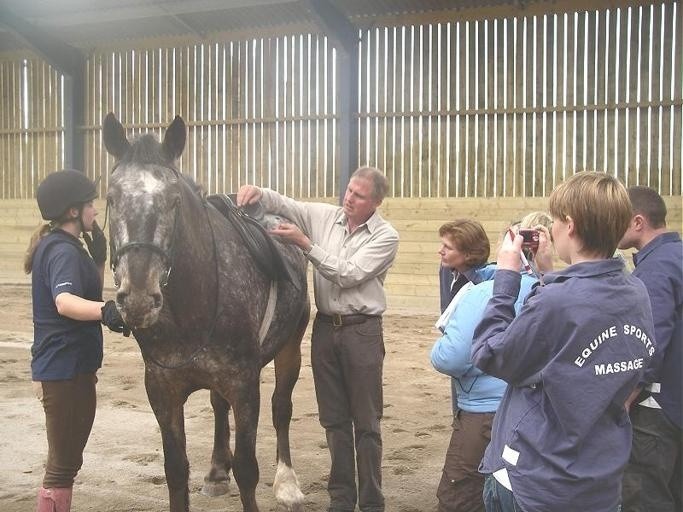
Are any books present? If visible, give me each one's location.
[435,280,474,333]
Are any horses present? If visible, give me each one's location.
[96,107,313,512]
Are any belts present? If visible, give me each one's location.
[317,312,370,327]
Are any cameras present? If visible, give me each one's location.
[516,229,542,246]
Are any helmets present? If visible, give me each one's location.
[38,169,100,220]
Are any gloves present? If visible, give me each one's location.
[101,299,132,337]
[83,220,108,264]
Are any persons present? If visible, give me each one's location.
[469,171,655,511]
[435,218,490,418]
[428,210,553,510]
[615,185,682,511]
[235,166,399,511]
[23,169,125,511]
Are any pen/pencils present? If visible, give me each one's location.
[509,229,533,275]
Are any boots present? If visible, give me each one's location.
[37,486,73,512]
